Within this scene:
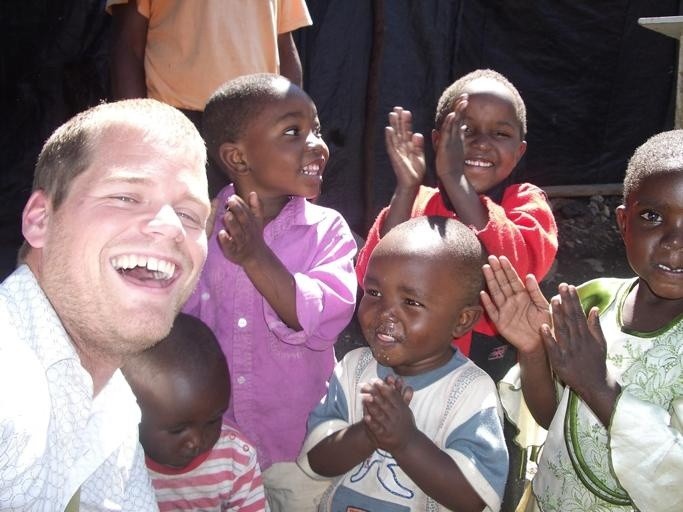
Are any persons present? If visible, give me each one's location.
[476,128,683,512]
[119,310,272,511]
[0,96,214,511]
[178,71,358,512]
[294,213,513,512]
[354,67,560,512]
[104,0,316,201]
